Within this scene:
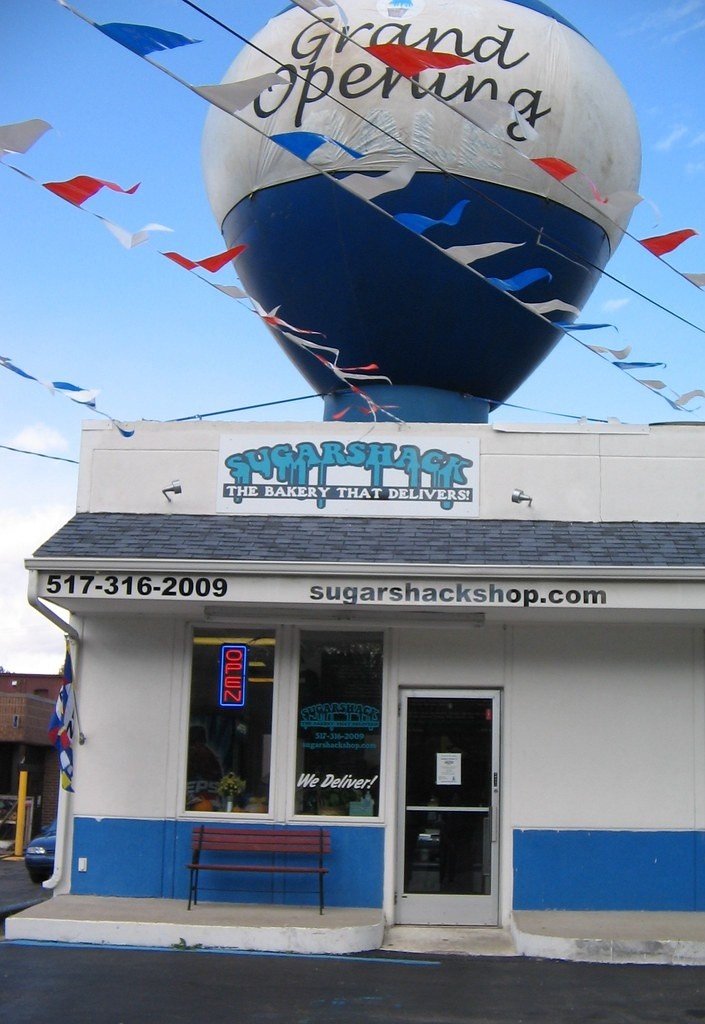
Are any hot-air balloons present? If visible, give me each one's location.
[202,0,645,424]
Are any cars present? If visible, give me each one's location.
[24,816,57,884]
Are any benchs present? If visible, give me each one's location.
[184,825,331,915]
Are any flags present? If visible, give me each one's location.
[48,647,76,794]
